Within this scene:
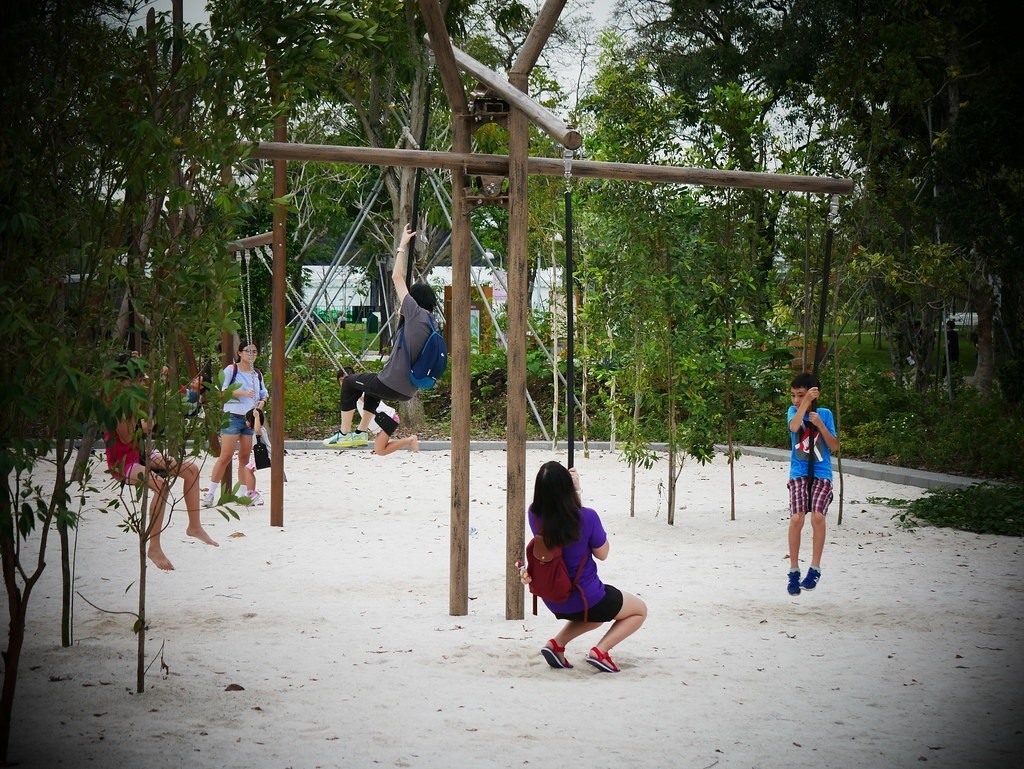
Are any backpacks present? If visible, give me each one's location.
[526,514,591,624]
[400,314,448,391]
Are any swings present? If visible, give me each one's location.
[253,245,399,437]
[235,249,272,470]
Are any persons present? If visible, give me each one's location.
[103,353,219,570]
[338,368,419,456]
[528,461,648,673]
[200,341,269,507]
[74,367,211,456]
[787,373,840,596]
[938,319,959,381]
[235,408,271,506]
[323,223,438,448]
[336,313,347,334]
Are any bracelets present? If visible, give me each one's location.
[395,248,405,255]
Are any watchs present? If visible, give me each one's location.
[576,488,583,494]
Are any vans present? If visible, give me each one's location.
[942,312,978,325]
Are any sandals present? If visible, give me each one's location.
[585,647,620,673]
[541,638,573,668]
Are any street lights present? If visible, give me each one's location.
[483,248,502,269]
[537,233,563,311]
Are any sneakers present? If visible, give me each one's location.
[322,430,353,447]
[254,496,264,505]
[244,491,260,507]
[352,432,368,446]
[799,567,821,591]
[786,570,801,596]
[201,493,214,507]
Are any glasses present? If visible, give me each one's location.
[243,349,258,353]
